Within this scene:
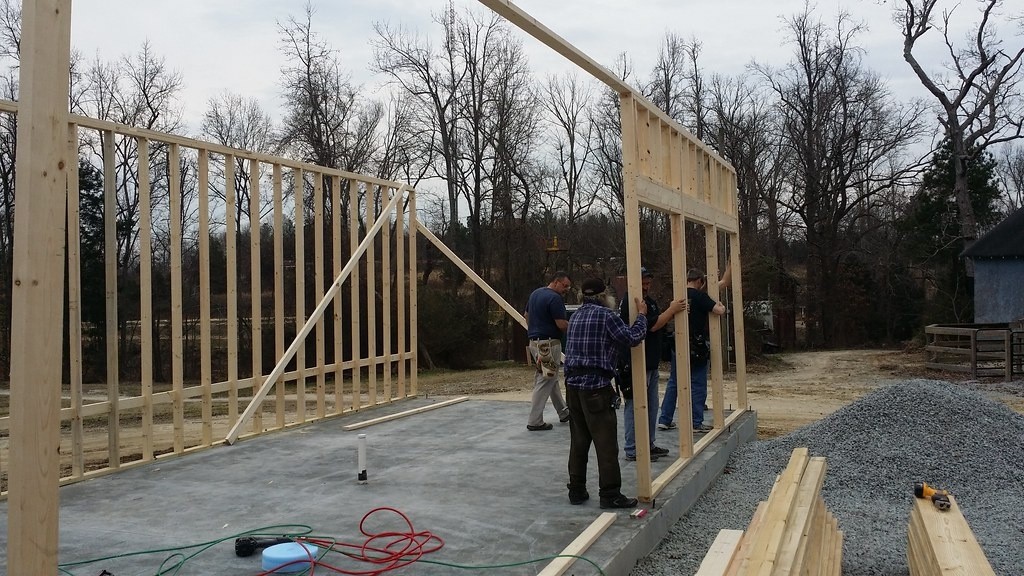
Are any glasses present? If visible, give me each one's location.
[557,278,571,292]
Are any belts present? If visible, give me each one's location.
[530,336,560,340]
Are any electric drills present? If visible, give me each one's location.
[915,482,950,511]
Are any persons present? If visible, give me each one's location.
[525,272,573,431]
[564,277,647,509]
[616,257,732,461]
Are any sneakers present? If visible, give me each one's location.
[599,493,638,509]
[693,423,714,433]
[658,421,677,429]
[650,446,669,455]
[626,452,659,462]
[569,490,589,504]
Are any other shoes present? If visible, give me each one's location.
[527,424,553,430]
[704,404,708,411]
[559,416,570,422]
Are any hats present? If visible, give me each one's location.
[582,277,606,296]
[641,267,654,279]
